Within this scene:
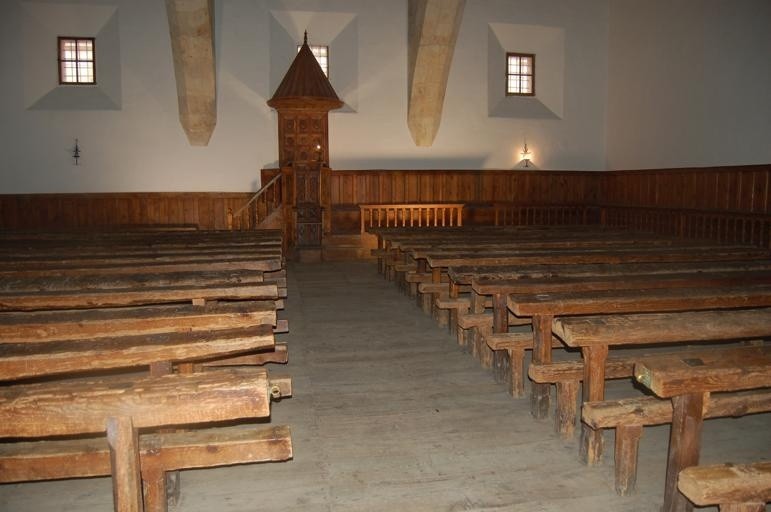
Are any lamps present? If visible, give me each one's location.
[520,143,532,167]
[72,139,81,166]
